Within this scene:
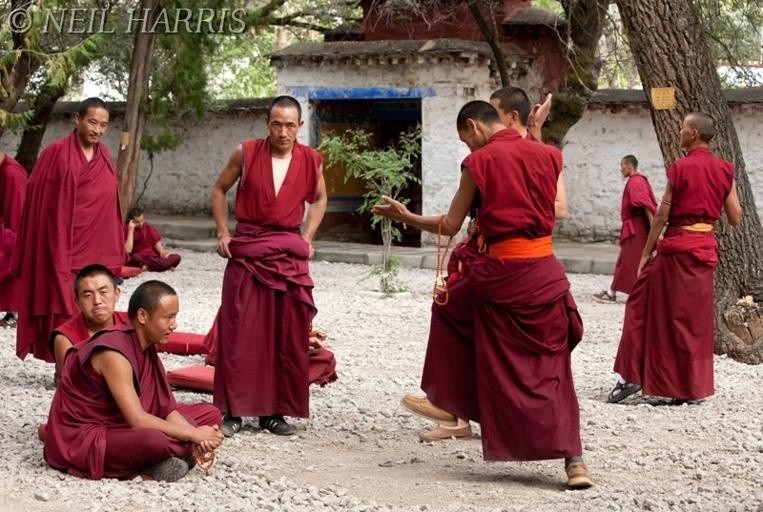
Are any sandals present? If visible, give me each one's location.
[609,379,641,402]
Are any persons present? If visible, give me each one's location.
[211,96,327,440]
[593,154,660,304]
[419,87,538,444]
[608,115,743,404]
[368,94,593,487]
[118,209,181,272]
[0,153,29,325]
[53,265,131,401]
[42,281,223,481]
[9,98,127,385]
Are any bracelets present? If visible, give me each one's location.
[188,442,216,470]
[433,215,458,305]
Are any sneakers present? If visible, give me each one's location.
[1,312,18,328]
[400,395,457,426]
[146,457,188,482]
[565,463,594,487]
[220,416,243,437]
[419,424,471,441]
[259,414,294,435]
[592,289,617,304]
